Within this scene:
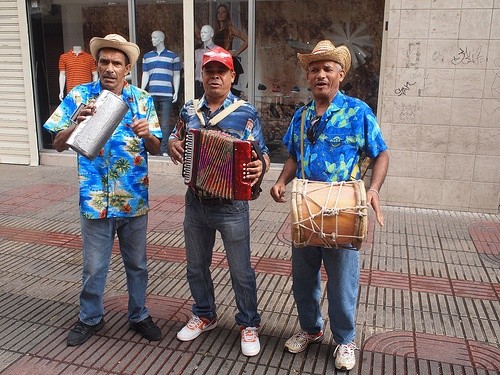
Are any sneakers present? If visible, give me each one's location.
[283,329,324,354]
[240,324,261,357]
[66,317,106,346]
[332,340,358,371]
[176,314,219,342]
[129,314,163,341]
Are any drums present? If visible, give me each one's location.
[289,176,368,251]
[65,89,130,162]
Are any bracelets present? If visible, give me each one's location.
[368,189,380,198]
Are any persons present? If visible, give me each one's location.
[141,30,181,156]
[194,25,221,99]
[59,46,98,101]
[44,33,164,346]
[270,40,389,370]
[212,4,248,92]
[168,47,269,356]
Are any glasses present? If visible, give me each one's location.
[306,115,323,144]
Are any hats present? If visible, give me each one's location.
[296,40,352,72]
[89,34,140,71]
[201,46,235,71]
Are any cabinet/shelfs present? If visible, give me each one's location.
[255,90,314,145]
